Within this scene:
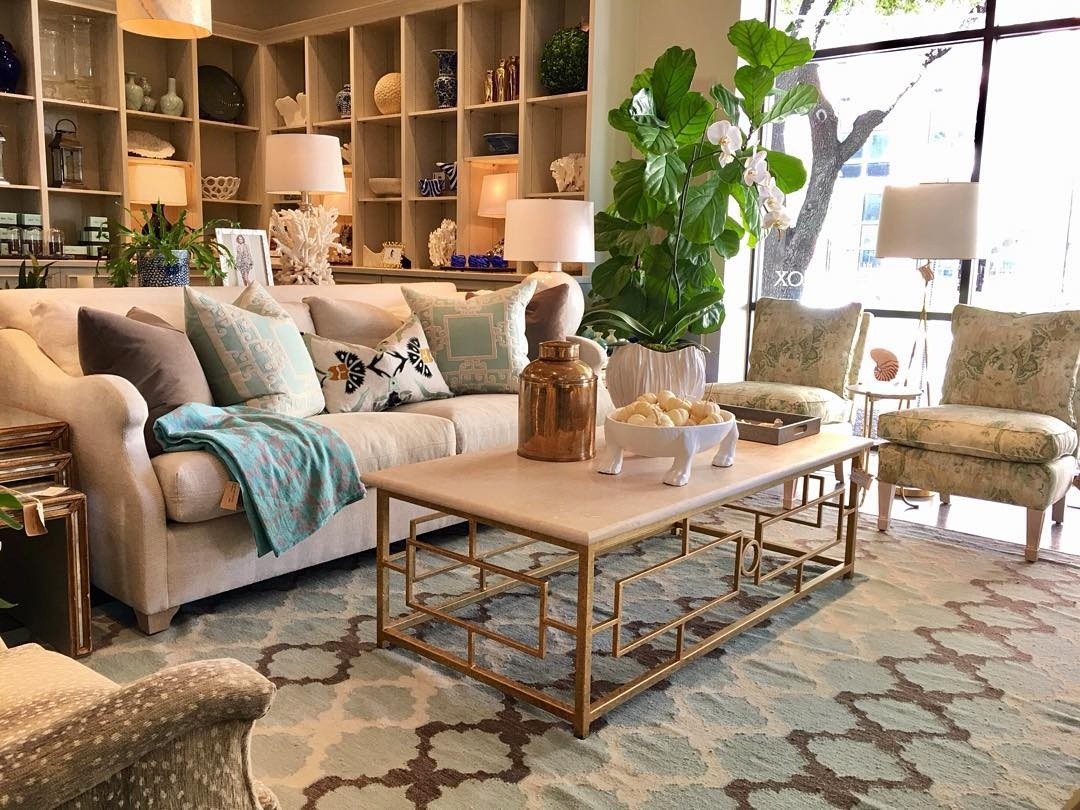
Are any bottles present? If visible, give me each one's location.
[430,49,457,110]
[484,56,519,103]
[518,339,597,462]
[0,34,19,94]
[126,71,185,117]
[335,84,351,119]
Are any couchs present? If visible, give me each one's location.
[0,639,281,810]
[0,281,618,636]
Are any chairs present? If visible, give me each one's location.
[876,305,1080,562]
[699,293,873,512]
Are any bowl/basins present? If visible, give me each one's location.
[597,407,740,485]
[368,178,402,197]
[482,133,519,154]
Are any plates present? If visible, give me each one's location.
[198,65,244,122]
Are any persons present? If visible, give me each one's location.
[235,235,254,287]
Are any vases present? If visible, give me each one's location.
[431,48,458,109]
[605,341,706,404]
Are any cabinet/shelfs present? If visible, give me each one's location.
[0,0,639,290]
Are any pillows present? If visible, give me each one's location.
[30,280,571,460]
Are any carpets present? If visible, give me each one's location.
[0,492,1080,810]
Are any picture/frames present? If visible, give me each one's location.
[215,228,275,287]
[378,241,406,269]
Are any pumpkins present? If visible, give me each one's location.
[613,390,724,427]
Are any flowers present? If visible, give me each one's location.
[575,108,792,354]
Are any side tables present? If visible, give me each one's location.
[0,405,92,660]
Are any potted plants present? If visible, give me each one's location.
[95,197,241,287]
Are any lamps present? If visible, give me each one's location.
[266,134,346,212]
[127,165,187,240]
[478,171,517,219]
[116,0,213,40]
[50,119,88,190]
[502,200,596,336]
[874,182,982,498]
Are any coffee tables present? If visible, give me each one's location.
[361,425,874,740]
[844,383,923,509]
